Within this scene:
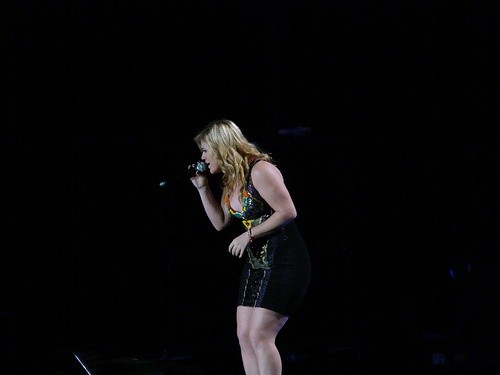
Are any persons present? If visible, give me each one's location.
[188,119,311,375]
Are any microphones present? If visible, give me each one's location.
[172,161,207,180]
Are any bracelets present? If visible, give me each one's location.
[247,228,255,242]
